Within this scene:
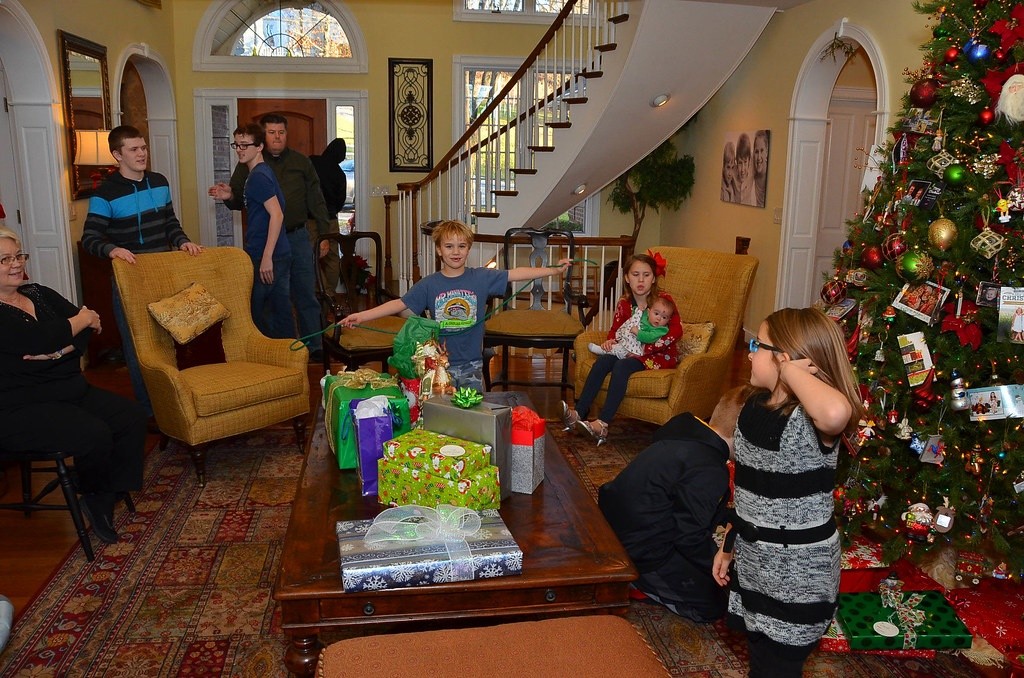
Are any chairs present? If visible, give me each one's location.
[110,246,311,488]
[0,445,135,563]
[572,246,759,426]
[483,227,585,392]
[315,231,427,376]
[558,245,599,297]
[313,615,673,678]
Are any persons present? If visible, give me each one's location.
[932,131,943,150]
[979,289,997,306]
[904,285,938,314]
[82,125,205,432]
[1011,308,1024,342]
[234,124,294,338]
[975,392,998,414]
[337,221,574,391]
[557,254,683,446]
[588,298,674,360]
[903,185,923,206]
[0,226,144,542]
[209,114,330,362]
[308,137,346,303]
[712,307,862,678]
[599,386,751,621]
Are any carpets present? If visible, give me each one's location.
[0,416,991,678]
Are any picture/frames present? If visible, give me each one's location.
[388,57,433,173]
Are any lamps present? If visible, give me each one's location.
[73,129,119,186]
[649,92,671,108]
[570,183,587,196]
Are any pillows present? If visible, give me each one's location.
[677,321,716,361]
[147,281,232,344]
[173,319,227,370]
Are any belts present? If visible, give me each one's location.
[285,220,309,234]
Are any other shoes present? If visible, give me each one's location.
[145,413,160,434]
[309,349,326,363]
[628,584,647,600]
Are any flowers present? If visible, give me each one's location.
[339,253,373,293]
[648,249,667,278]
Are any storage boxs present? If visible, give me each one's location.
[818,590,973,659]
[324,373,524,593]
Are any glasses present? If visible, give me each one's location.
[230,142,258,150]
[0,253,30,266]
[750,338,784,354]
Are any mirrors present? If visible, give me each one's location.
[56,27,120,202]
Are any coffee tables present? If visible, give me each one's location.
[273,391,641,678]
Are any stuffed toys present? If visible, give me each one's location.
[999,74,1024,185]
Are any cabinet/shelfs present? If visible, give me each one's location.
[76,241,122,369]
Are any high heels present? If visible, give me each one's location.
[556,400,582,432]
[574,419,609,447]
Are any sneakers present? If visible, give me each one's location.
[79,493,117,543]
